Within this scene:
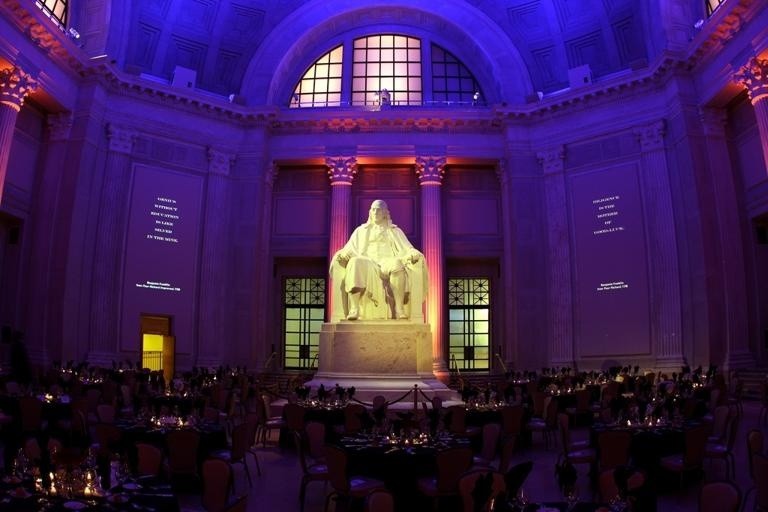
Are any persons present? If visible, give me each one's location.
[1,355,767,511]
[335,198,423,318]
